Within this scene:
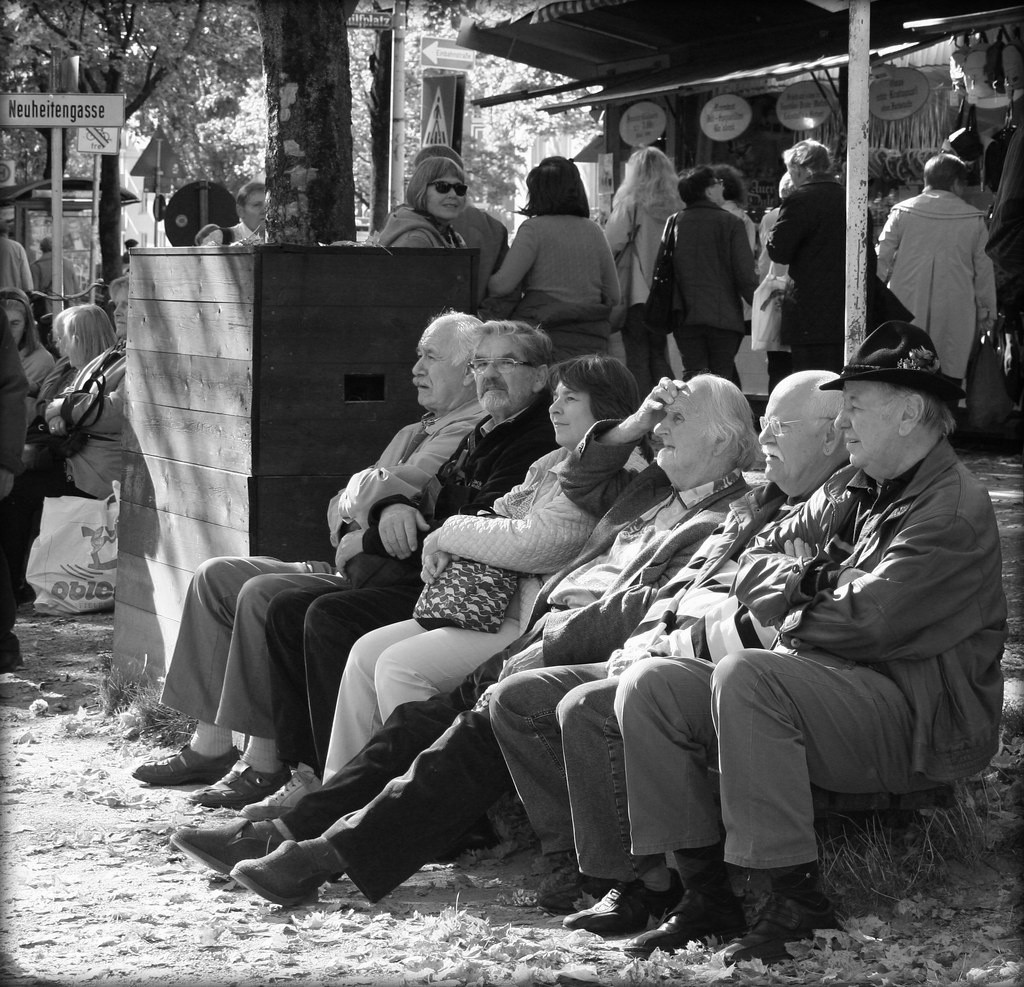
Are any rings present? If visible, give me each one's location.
[51,427,55,429]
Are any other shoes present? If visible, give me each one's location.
[230,840,316,904]
[562,868,684,935]
[536,866,628,917]
[170,820,280,876]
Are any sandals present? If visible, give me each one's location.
[187,760,292,812]
[132,743,241,785]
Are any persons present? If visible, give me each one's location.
[133,312,486,808]
[490,370,851,935]
[200,181,267,244]
[382,121,1024,453]
[241,320,559,826]
[624,320,1007,966]
[0,220,137,673]
[171,373,757,911]
[323,353,650,793]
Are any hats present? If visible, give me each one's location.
[820,321,967,400]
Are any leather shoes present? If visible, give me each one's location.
[624,888,748,961]
[724,891,844,967]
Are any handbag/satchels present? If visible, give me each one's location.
[25,481,121,618]
[602,205,641,334]
[641,211,679,335]
[16,371,106,492]
[413,504,545,634]
[967,328,1015,432]
[751,260,795,352]
[341,449,472,589]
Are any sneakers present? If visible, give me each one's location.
[241,771,322,822]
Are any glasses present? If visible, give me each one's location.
[711,179,724,187]
[427,181,467,197]
[760,416,836,437]
